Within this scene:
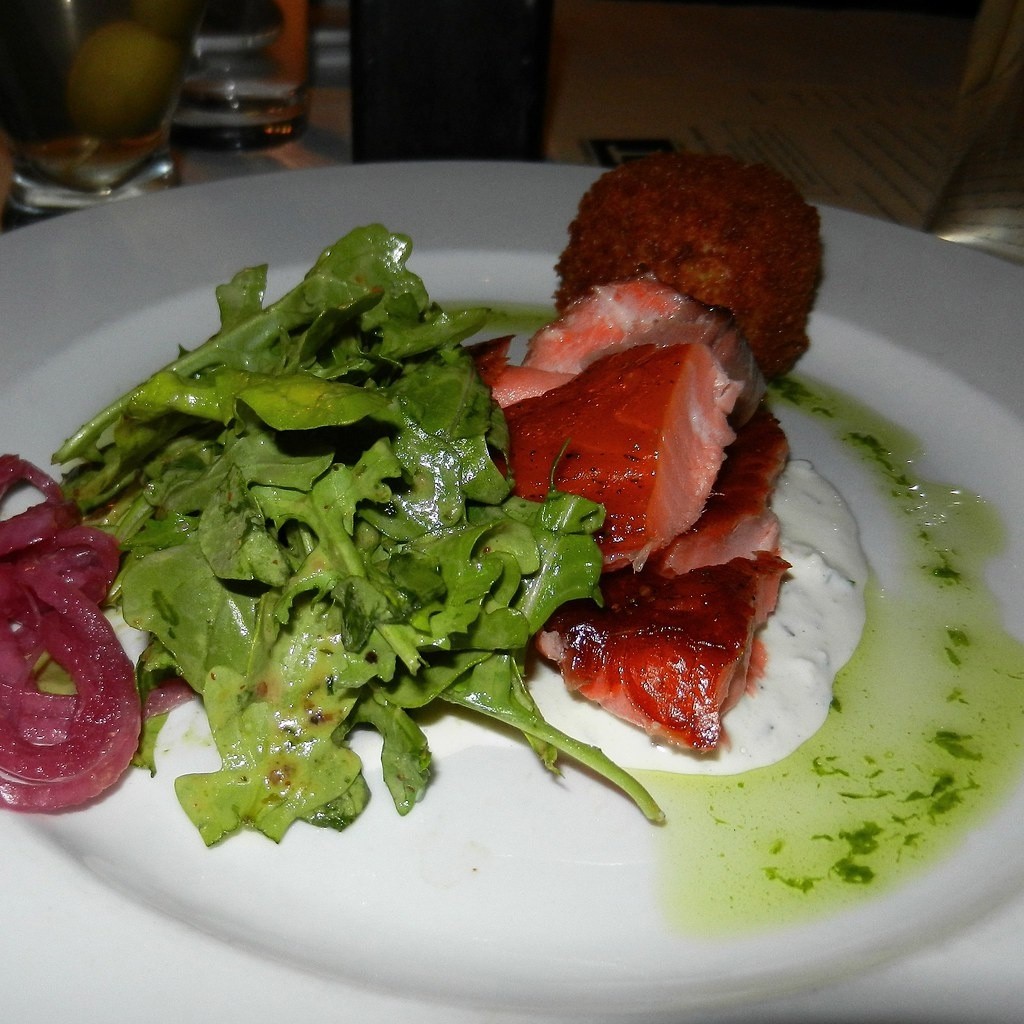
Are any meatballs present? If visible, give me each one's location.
[557,155,824,383]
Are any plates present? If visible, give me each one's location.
[0,153,1022,1022]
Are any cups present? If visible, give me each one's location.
[162,0,313,150]
[0,0,209,216]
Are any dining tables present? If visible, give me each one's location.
[0,88,1020,1021]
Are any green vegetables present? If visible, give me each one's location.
[31,220,668,844]
